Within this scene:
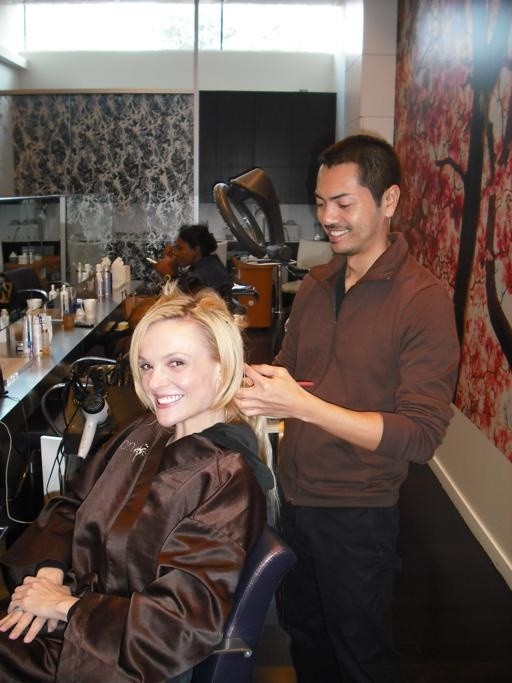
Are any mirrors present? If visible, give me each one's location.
[2,197,68,332]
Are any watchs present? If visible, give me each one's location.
[162,272,177,284]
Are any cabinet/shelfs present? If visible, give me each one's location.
[200,92,336,202]
[233,257,275,327]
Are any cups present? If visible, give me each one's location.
[84,299,97,317]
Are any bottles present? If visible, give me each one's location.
[60,284,70,313]
[75,297,84,322]
[95,264,113,297]
[49,281,60,302]
[9,246,42,266]
[63,311,74,331]
[26,298,43,310]
[25,309,53,356]
[76,261,94,282]
[1,308,10,329]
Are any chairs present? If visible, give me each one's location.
[11,355,118,506]
[193,520,299,683]
[272,238,335,352]
[230,282,261,314]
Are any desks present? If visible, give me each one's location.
[1,280,145,423]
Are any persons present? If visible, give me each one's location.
[1,278,280,681]
[233,133,460,681]
[100,221,237,363]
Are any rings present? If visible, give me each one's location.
[14,603,27,615]
